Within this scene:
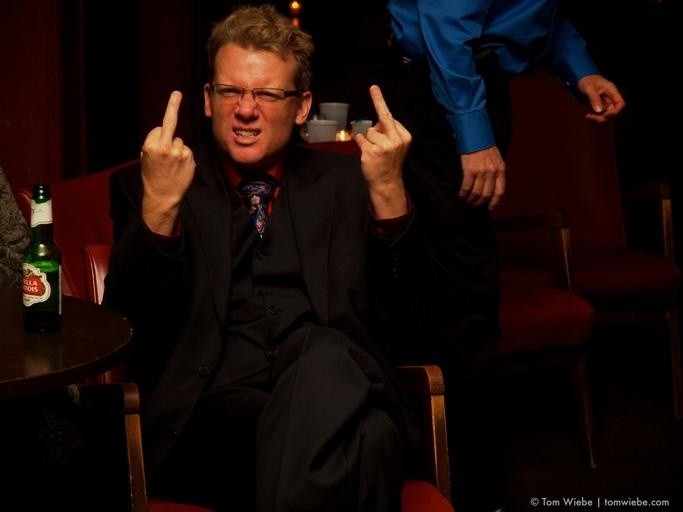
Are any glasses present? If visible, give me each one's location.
[209,84,301,103]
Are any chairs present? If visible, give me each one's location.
[440,72,683,475]
[82,237,456,512]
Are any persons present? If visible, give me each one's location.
[103,0,416,510]
[0,168,36,289]
[369,0,631,510]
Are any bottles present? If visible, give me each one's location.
[20,182,62,331]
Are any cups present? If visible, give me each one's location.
[304,119,338,144]
[317,102,349,130]
[350,120,371,139]
[299,111,326,142]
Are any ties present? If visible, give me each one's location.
[237,174,279,239]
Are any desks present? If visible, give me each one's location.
[1,281,136,512]
